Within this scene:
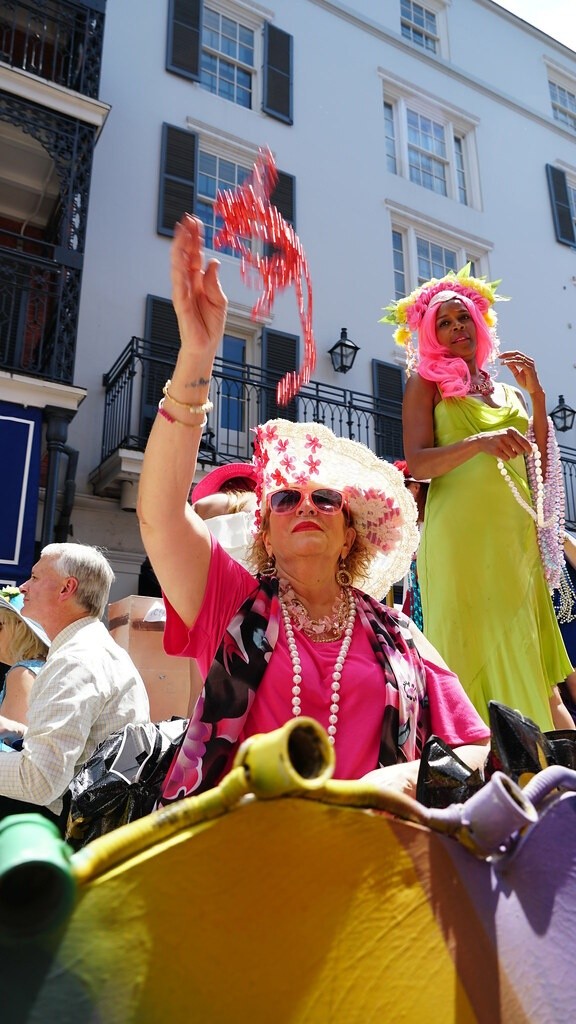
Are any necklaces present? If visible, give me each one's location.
[277,576,357,747]
[212,144,316,407]
[462,368,495,396]
[552,564,576,625]
[496,414,566,597]
[407,560,424,632]
[278,574,351,644]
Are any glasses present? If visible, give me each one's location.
[265,488,350,525]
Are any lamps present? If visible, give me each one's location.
[549,394,576,433]
[329,327,360,376]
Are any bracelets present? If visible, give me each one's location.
[158,398,208,429]
[162,379,214,415]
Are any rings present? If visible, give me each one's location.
[522,357,526,362]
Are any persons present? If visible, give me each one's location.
[550,529,576,705]
[0,539,151,841]
[135,211,493,815]
[0,585,53,752]
[391,459,433,633]
[190,463,260,522]
[377,261,576,802]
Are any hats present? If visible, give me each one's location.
[192,464,258,503]
[253,419,420,601]
[0,587,51,648]
